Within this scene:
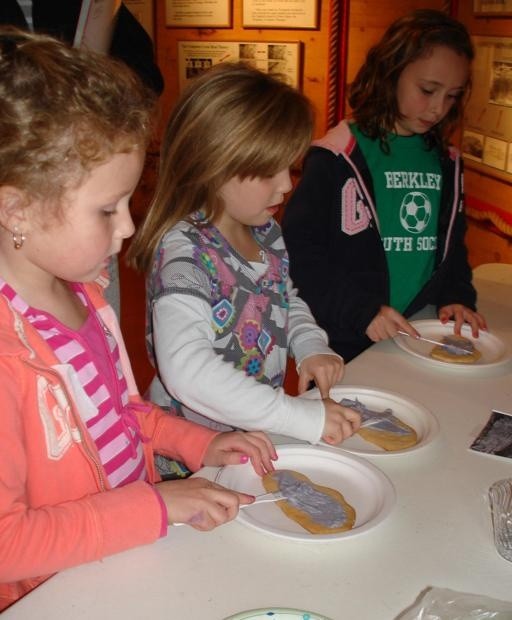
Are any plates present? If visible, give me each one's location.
[292,379,442,461]
[391,318,511,371]
[207,440,403,543]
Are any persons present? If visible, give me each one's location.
[0,24,278,613]
[1,0,166,330]
[281,8,489,392]
[122,60,366,481]
